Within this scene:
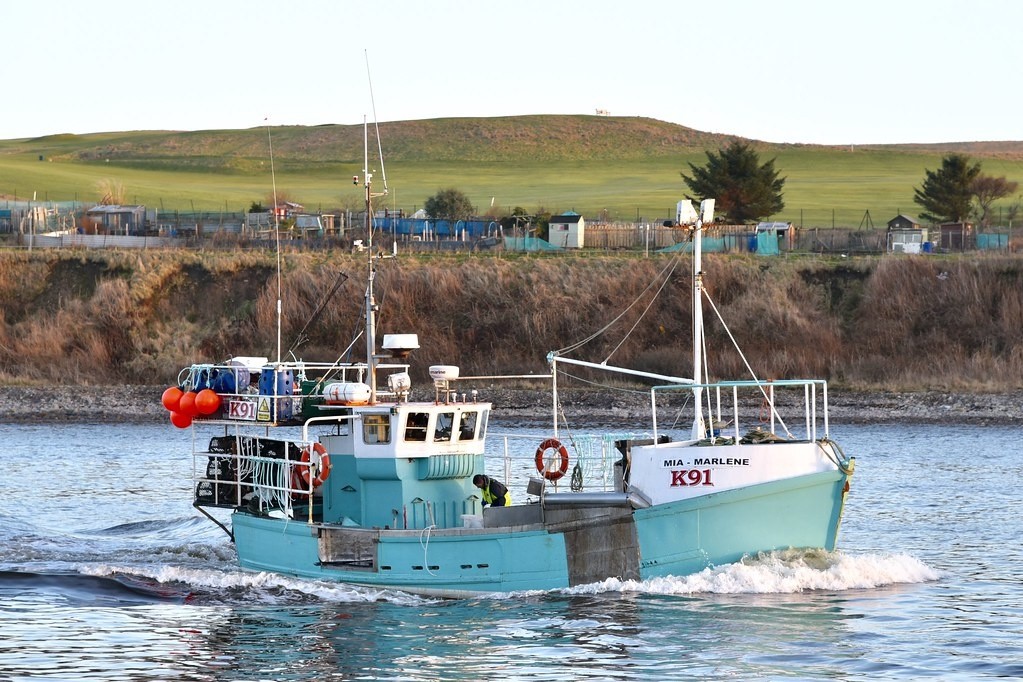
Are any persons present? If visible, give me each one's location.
[406,413,428,438]
[459,419,468,440]
[473,474,511,507]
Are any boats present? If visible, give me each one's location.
[162,49,855,600]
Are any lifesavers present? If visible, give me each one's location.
[535,438,569,481]
[300,442,330,486]
